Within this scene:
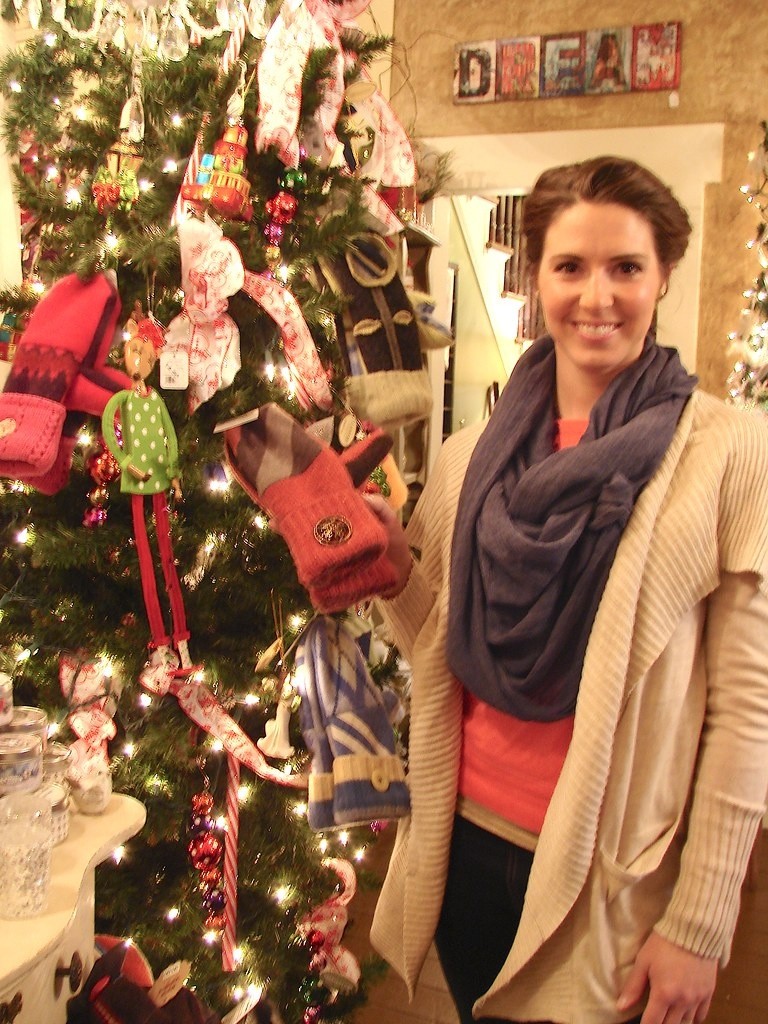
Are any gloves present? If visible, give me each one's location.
[224,400,401,616]
[0,269,133,495]
[296,617,411,832]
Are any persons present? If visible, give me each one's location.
[360,157,768,1024]
[103,302,194,671]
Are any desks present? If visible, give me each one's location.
[0,793,148,1024]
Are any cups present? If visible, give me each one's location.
[0,793,53,920]
